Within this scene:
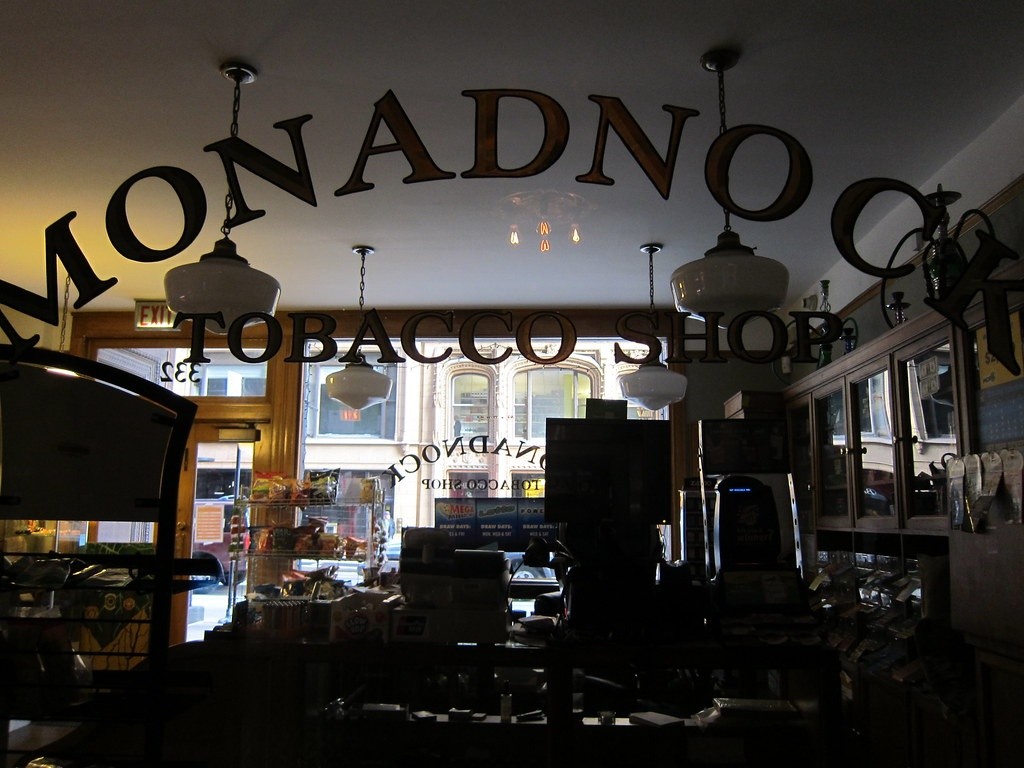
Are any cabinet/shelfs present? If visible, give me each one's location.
[208,622,824,768]
[778,255,1024,767]
[232,478,386,603]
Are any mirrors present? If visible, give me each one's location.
[852,370,896,518]
[816,388,849,518]
[792,192,1024,382]
[907,342,958,515]
[791,402,814,533]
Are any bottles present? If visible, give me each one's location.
[262,600,313,642]
[500,680,513,723]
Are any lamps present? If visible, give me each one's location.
[163,62,280,335]
[815,279,832,370]
[326,246,392,409]
[924,183,970,310]
[620,243,688,411]
[670,48,789,330]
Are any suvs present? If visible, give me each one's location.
[194,498,248,595]
